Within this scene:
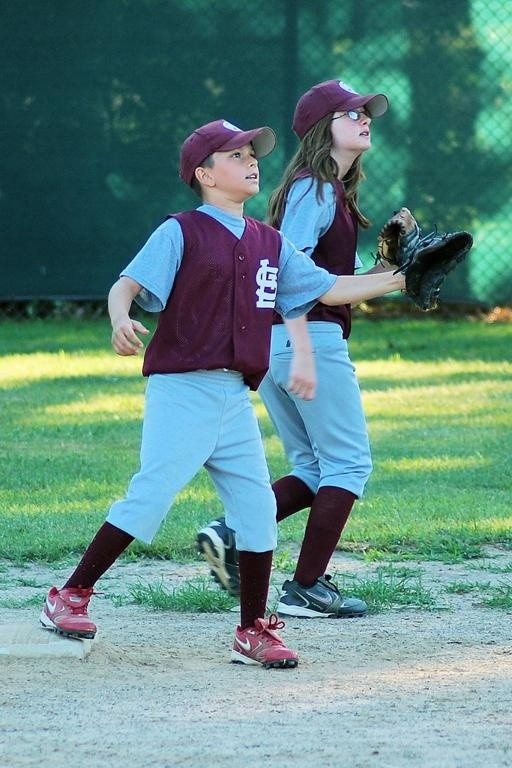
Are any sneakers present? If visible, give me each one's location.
[38,587,97,640]
[276,575,367,618]
[230,618,298,668]
[196,517,241,597]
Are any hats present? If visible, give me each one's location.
[292,79,390,140]
[180,119,277,186]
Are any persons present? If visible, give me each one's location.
[39,119,407,670]
[198,78,400,617]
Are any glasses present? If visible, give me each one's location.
[331,109,370,121]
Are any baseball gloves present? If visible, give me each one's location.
[404,232,473,311]
[378,207,422,267]
